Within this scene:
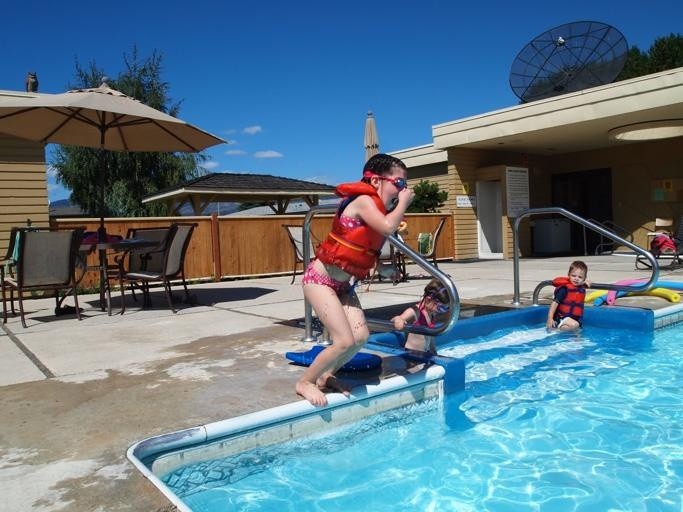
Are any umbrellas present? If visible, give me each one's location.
[1,77,229,312]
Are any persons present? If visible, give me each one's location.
[389,280,450,354]
[650,230,675,257]
[394,217,409,284]
[548,260,590,333]
[295,153,417,407]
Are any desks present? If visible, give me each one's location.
[55,240,160,317]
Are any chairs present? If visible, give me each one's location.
[6,227,88,326]
[106,227,170,309]
[646,217,675,251]
[399,217,447,283]
[376,235,404,282]
[117,222,198,314]
[281,223,322,285]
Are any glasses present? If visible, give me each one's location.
[436,304,450,315]
[382,177,410,188]
